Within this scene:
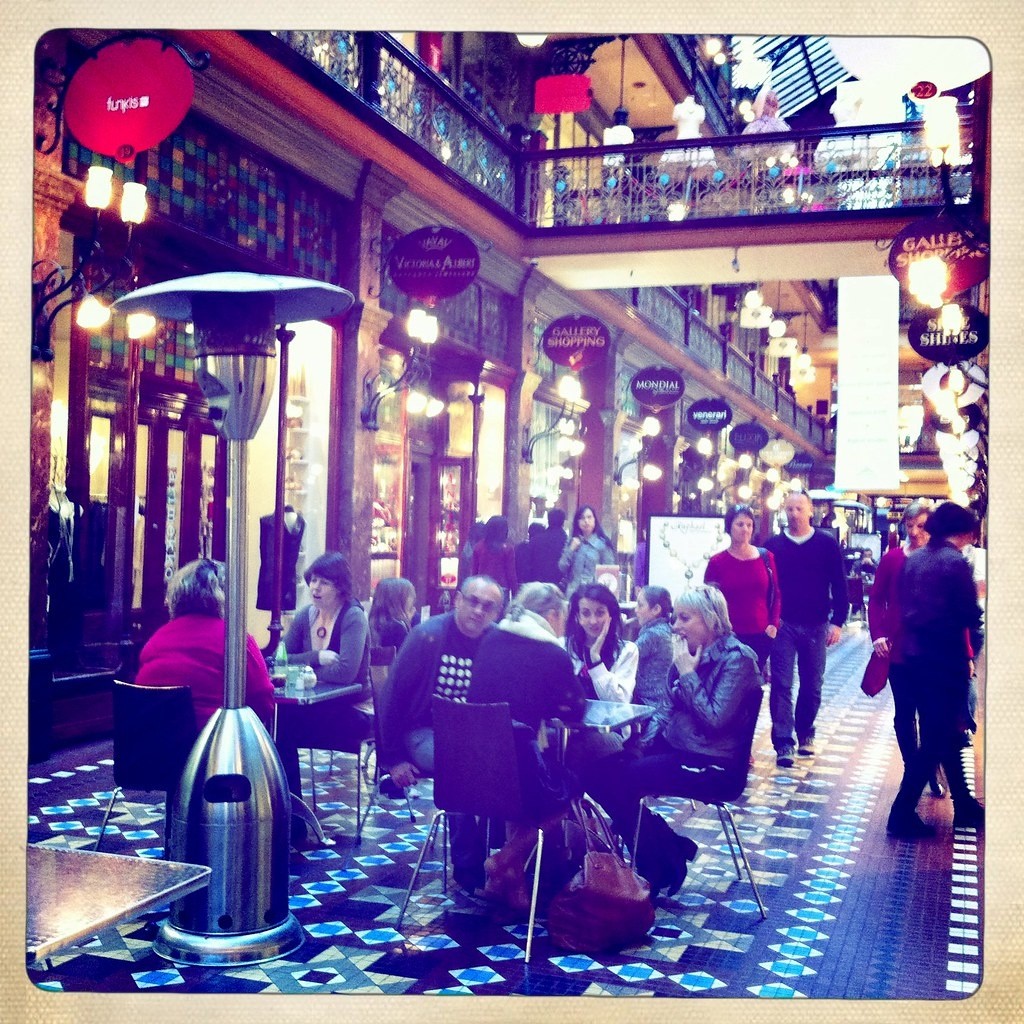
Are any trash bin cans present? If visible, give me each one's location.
[152,708,305,966]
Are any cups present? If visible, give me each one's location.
[272,674,287,696]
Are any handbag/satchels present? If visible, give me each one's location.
[546,797,657,952]
[860,651,891,697]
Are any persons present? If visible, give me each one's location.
[131,486,986,898]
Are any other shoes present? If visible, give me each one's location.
[797,735,815,755]
[953,798,985,826]
[885,812,936,839]
[379,770,408,799]
[777,744,795,765]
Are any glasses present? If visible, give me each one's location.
[734,504,750,512]
[457,588,503,612]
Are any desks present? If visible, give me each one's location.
[27,846,213,969]
[267,672,362,847]
[509,697,657,857]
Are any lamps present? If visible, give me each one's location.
[358,33,990,508]
[32,166,156,359]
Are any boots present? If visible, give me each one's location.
[636,812,698,898]
[484,807,570,909]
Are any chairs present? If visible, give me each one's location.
[94,547,873,966]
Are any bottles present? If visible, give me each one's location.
[273,638,317,690]
